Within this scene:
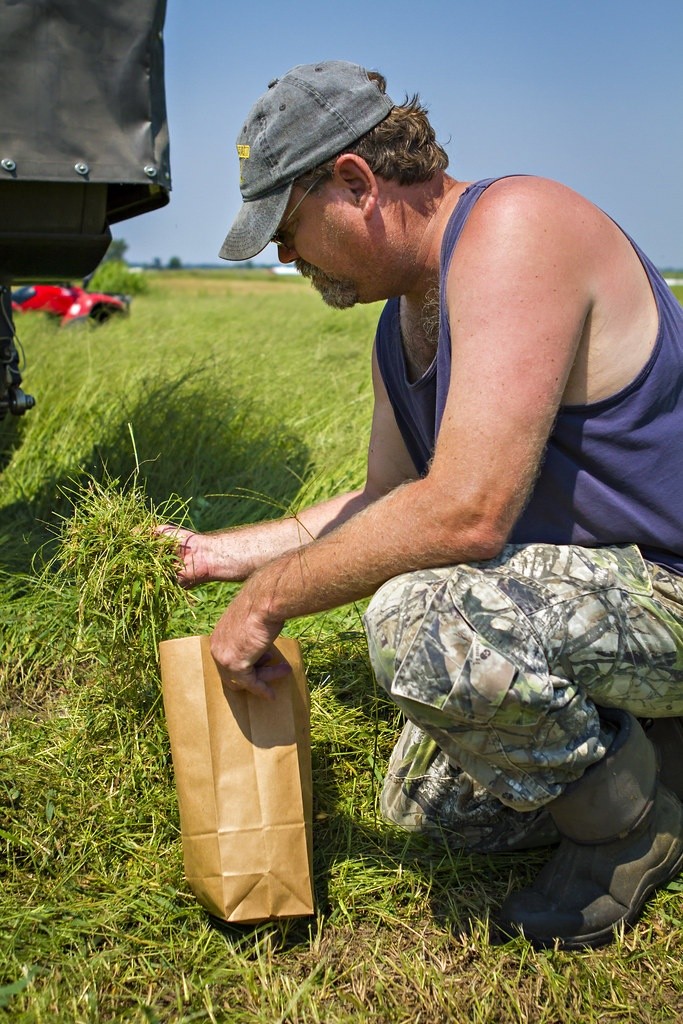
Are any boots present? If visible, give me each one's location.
[501,710,683,946]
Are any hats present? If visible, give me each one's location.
[218,60,395,261]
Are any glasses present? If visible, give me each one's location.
[270,174,328,251]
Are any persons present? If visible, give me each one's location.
[128,56,678,955]
